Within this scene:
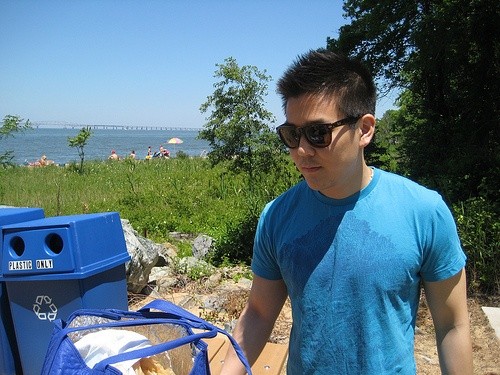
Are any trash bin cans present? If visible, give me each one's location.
[1,207,44,374]
[0,211,132,374]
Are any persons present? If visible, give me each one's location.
[219,46,474,375]
[108,150,119,159]
[40,155,49,166]
[130,150,136,158]
[145,145,170,159]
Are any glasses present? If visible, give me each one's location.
[277,116,358,148]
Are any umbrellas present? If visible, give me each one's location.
[168,137,184,144]
